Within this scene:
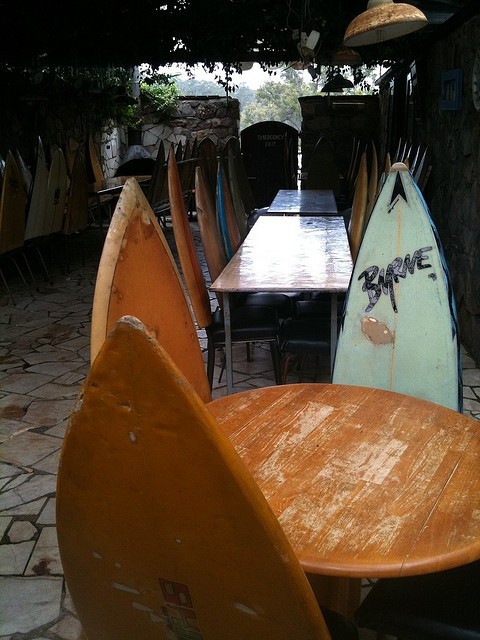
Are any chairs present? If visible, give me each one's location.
[87,132,122,223]
[87,177,212,409]
[345,153,366,262]
[0,135,70,305]
[57,315,330,638]
[192,164,293,362]
[334,159,463,412]
[225,144,251,242]
[149,135,202,232]
[164,144,284,387]
[215,162,242,260]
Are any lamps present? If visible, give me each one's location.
[342,0,427,47]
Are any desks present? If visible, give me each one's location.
[266,188,338,214]
[198,381,479,638]
[212,215,354,396]
